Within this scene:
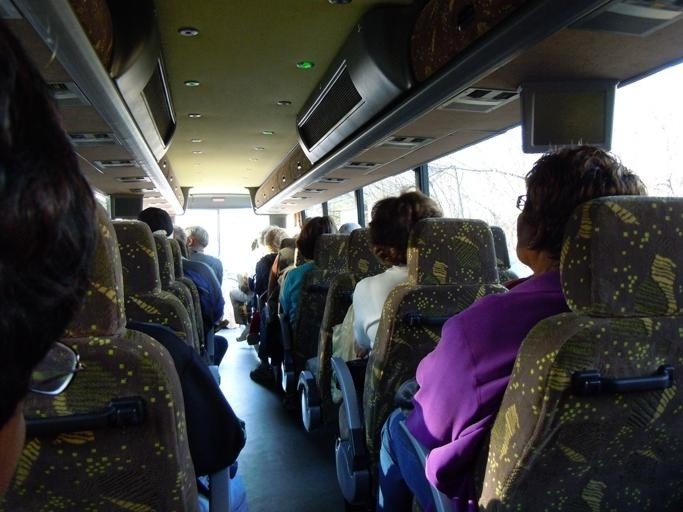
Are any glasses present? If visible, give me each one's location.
[29,340,86,396]
[516,196,530,211]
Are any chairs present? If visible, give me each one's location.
[244,194,683,511]
[1,196,229,512]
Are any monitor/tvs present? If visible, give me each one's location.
[270,215,286,228]
[522,79,614,153]
[111,194,143,219]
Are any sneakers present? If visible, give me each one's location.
[237,323,251,341]
[250,367,275,387]
[215,320,228,333]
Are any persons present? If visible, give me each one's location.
[352,185,442,397]
[137,207,227,366]
[229,216,362,390]
[0,15,102,503]
[126,323,248,512]
[372,140,648,512]
